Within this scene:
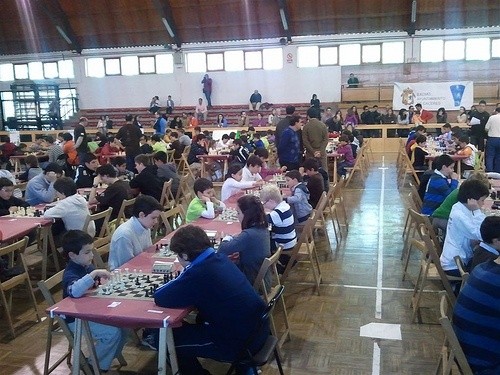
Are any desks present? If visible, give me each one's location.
[455,178,500,265]
[196,154,230,186]
[303,153,345,186]
[0,187,141,241]
[45,172,308,375]
[342,123,487,152]
[424,154,468,180]
[342,80,500,103]
[9,154,47,173]
[101,154,126,165]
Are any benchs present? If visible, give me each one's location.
[60,102,339,128]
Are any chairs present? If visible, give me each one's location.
[223,138,372,375]
[396,137,484,375]
[0,140,223,338]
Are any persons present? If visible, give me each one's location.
[96,108,227,230]
[108,195,164,350]
[196,196,271,325]
[347,74,362,104]
[43,177,96,257]
[452,255,500,375]
[406,123,500,241]
[89,164,128,237]
[361,100,476,138]
[153,224,270,375]
[196,97,209,124]
[57,230,129,375]
[166,95,174,114]
[216,105,364,224]
[149,95,159,116]
[260,185,298,275]
[185,178,225,224]
[452,216,500,298]
[248,90,262,112]
[0,98,101,218]
[439,176,489,296]
[201,74,212,107]
[310,94,320,106]
[466,100,500,173]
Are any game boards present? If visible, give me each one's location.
[256,181,275,189]
[45,196,61,206]
[244,190,265,204]
[87,273,179,301]
[27,152,46,156]
[208,144,235,155]
[268,173,287,181]
[324,138,340,154]
[213,208,241,221]
[152,239,228,259]
[422,134,458,158]
[78,194,89,203]
[449,178,500,217]
[1,207,45,218]
[96,172,138,189]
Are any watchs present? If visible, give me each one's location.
[93,184,98,189]
[126,174,130,178]
